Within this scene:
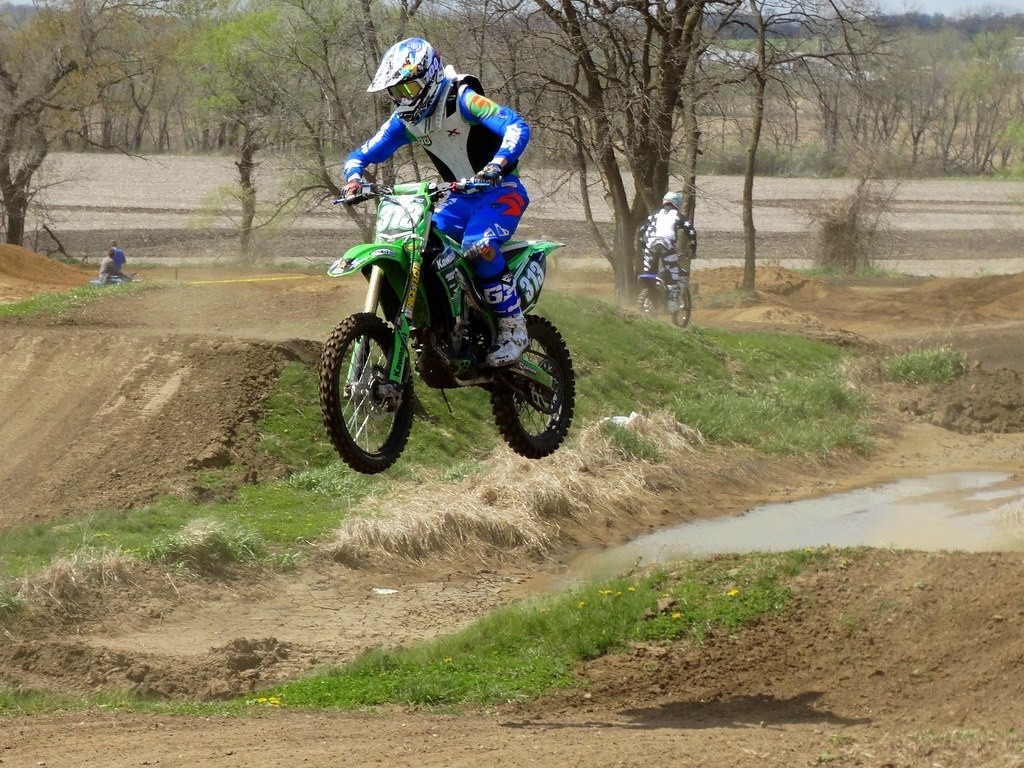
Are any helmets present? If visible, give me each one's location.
[366,37,448,126]
[662,191,682,209]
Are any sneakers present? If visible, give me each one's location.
[485,313,529,368]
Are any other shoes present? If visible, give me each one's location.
[668,300,682,312]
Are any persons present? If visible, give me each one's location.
[638,192,696,314]
[344,36,531,369]
[110,241,125,272]
[99,249,122,284]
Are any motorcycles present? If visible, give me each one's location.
[636,253,697,327]
[317,176,576,475]
[89,273,139,286]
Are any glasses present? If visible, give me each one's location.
[390,81,422,103]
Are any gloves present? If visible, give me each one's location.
[474,164,501,191]
[341,179,362,199]
